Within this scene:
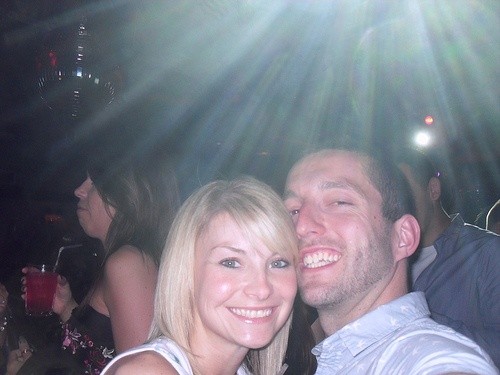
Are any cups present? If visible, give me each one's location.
[24,264,61,317]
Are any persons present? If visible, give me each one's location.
[390,147,500,374]
[275,137,500,375]
[1,179,102,375]
[20,145,182,375]
[96,171,300,375]
